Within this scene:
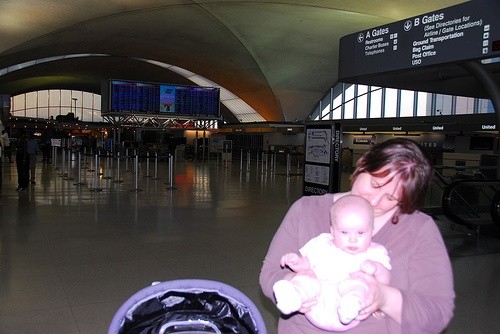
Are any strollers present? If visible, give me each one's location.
[107,278,269,334]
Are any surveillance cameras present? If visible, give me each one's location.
[437,109,442,116]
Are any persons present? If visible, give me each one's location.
[273,195,392,331]
[0,130,11,163]
[259,137,455,334]
[26,133,40,183]
[38,130,51,163]
[16,134,29,191]
